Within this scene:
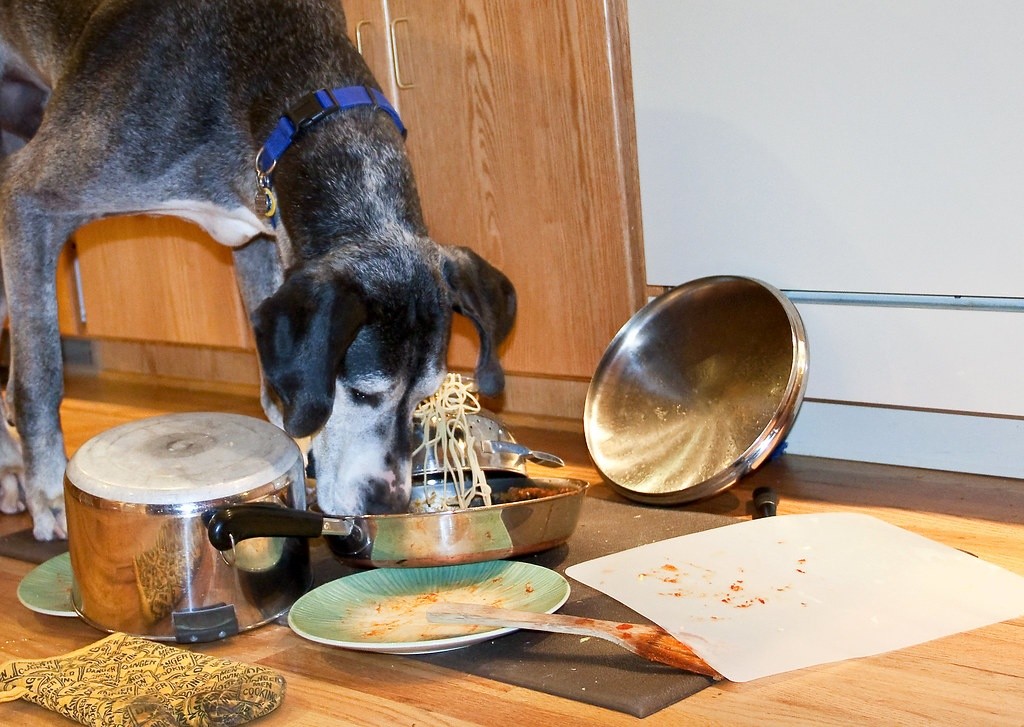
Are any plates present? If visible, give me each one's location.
[15,549,79,619]
[287,558,571,655]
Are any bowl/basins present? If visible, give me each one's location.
[581,274,810,509]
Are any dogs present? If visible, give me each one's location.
[1,0,518,545]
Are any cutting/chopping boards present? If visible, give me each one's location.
[278,494,754,720]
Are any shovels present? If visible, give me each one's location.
[427,600,726,681]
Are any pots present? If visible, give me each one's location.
[64,410,314,645]
[207,476,592,570]
